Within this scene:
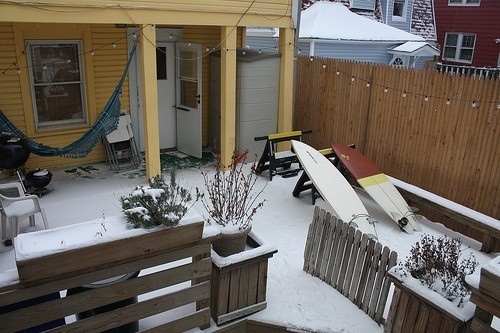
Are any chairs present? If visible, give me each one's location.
[101,108,142,173]
[0,181,50,247]
[0,133,33,193]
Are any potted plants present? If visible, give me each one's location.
[384,231,479,333]
[14,165,205,284]
[196,147,270,258]
